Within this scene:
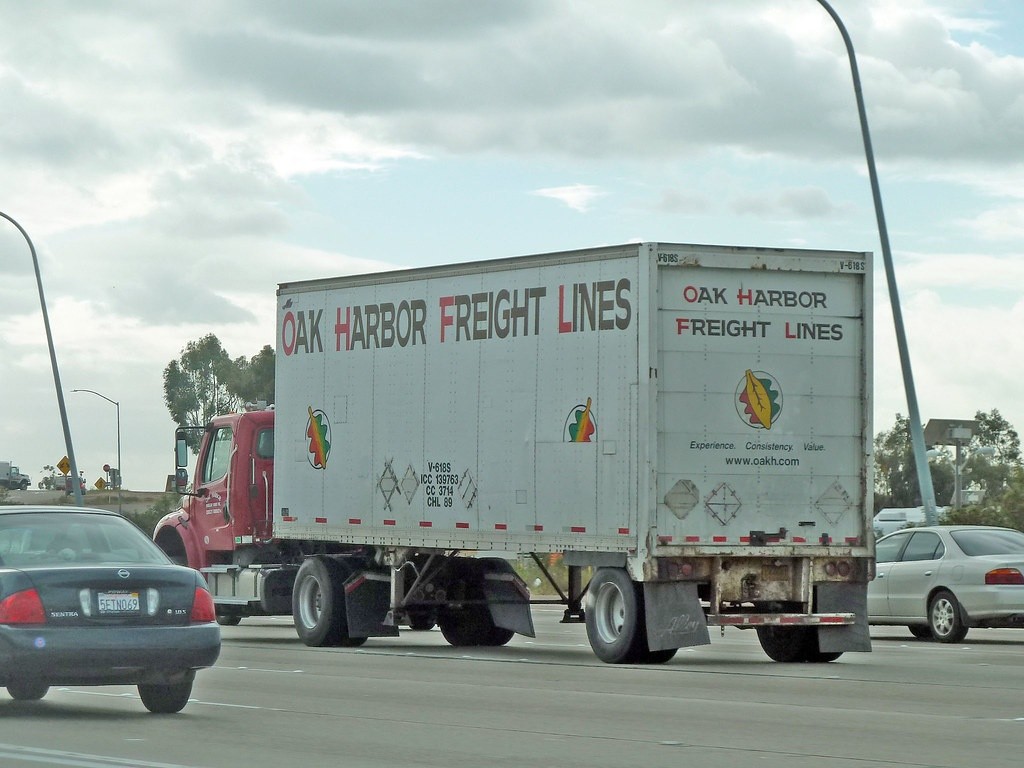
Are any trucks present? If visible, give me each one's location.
[0,461,31,490]
[153,242,876,664]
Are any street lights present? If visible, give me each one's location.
[927,445,995,509]
[70,389,121,514]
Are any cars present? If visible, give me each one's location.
[51,475,86,492]
[868,525,1024,643]
[0,505,221,713]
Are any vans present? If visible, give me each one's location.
[873,506,946,539]
